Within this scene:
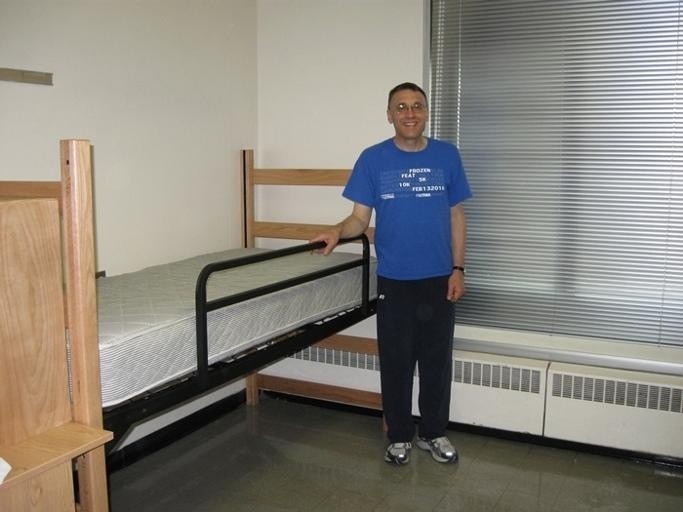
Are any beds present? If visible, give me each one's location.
[0,139,389,512]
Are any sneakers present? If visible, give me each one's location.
[416,431,458,464]
[384,440,414,465]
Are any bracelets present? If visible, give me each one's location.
[451,264,466,274]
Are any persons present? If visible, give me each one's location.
[306,83,474,468]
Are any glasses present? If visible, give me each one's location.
[391,102,427,112]
